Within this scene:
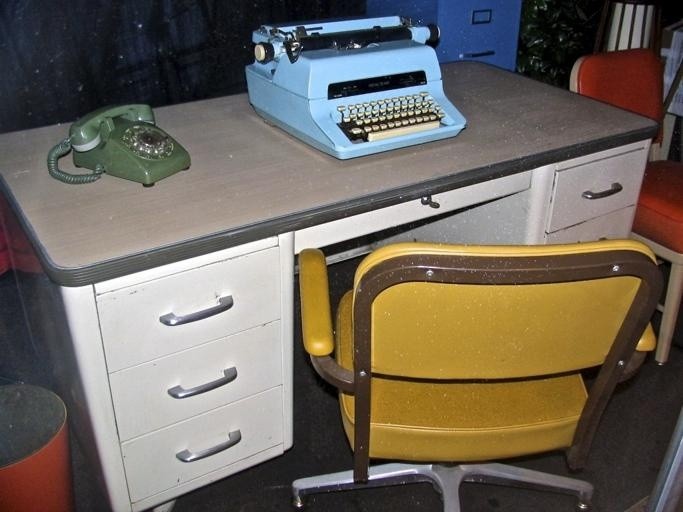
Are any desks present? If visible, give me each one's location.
[1,59,659,512]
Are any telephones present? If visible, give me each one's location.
[47,102,191,187]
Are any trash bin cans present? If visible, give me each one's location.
[0,384,72,512]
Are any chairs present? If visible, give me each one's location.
[569,47,683,366]
[291,240,663,512]
